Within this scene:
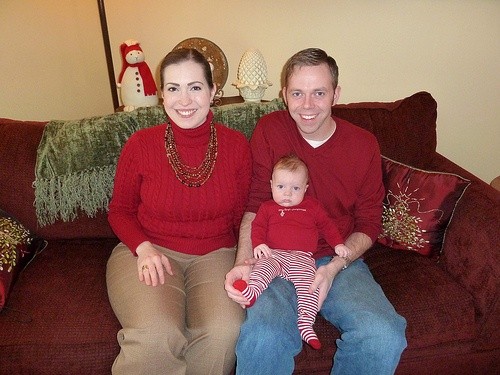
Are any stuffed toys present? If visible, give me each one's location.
[117,40,159,112]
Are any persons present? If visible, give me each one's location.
[106,48,252,375]
[233,154,354,350]
[224,47,409,375]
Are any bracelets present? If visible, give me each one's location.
[330,253,351,270]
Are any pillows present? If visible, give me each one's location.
[375,154,471,263]
[0,207,48,312]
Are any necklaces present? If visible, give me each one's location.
[165,119,218,187]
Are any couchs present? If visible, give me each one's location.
[0,90,500,375]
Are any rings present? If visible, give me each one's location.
[142,265,148,272]
